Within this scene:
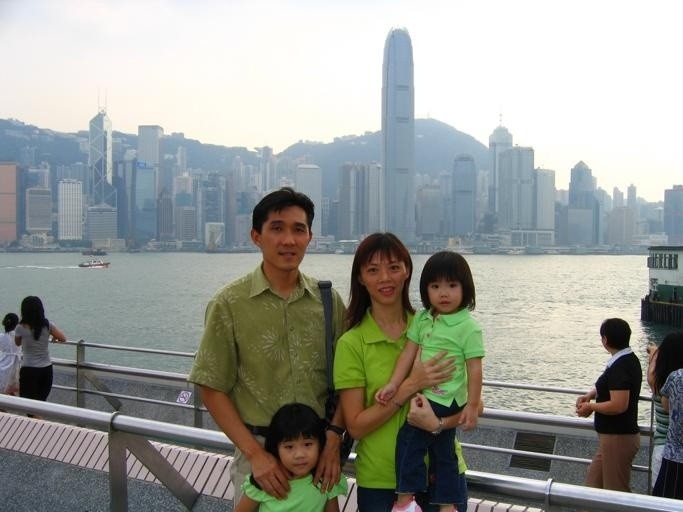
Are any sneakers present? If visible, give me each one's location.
[391,496,422,511]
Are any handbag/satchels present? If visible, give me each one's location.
[327,390,354,466]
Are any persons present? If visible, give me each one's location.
[576,318,642,493]
[187,188,348,512]
[0,313,21,394]
[375,250,486,512]
[15,296,65,421]
[232,404,347,512]
[647,331,683,501]
[333,231,486,512]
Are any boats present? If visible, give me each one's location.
[82,248,108,256]
[78,254,111,268]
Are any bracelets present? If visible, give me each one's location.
[432,418,442,433]
[391,398,403,408]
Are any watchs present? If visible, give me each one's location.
[327,424,347,444]
